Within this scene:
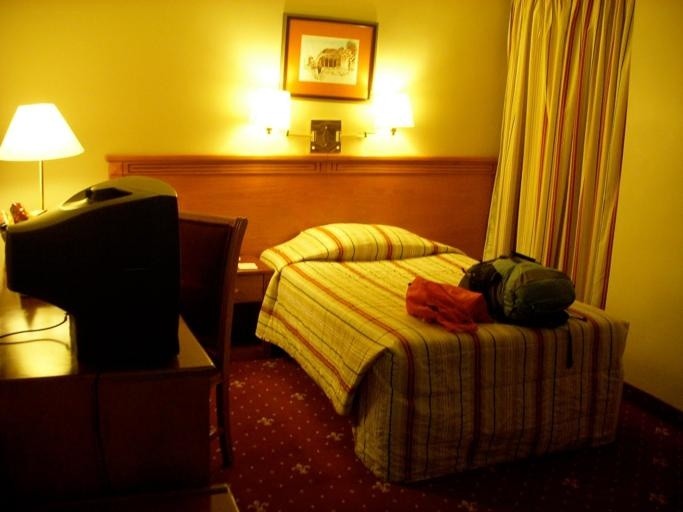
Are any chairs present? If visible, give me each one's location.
[176,210,249,469]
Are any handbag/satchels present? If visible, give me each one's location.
[406,276,493,332]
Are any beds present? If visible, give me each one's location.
[258,223,630,484]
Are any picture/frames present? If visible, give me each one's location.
[279,12,378,102]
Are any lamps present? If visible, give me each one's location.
[0,102,85,218]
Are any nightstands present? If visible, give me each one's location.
[229,254,275,363]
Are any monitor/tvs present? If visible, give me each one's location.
[6,174,179,373]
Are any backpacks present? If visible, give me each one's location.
[458,252,576,328]
